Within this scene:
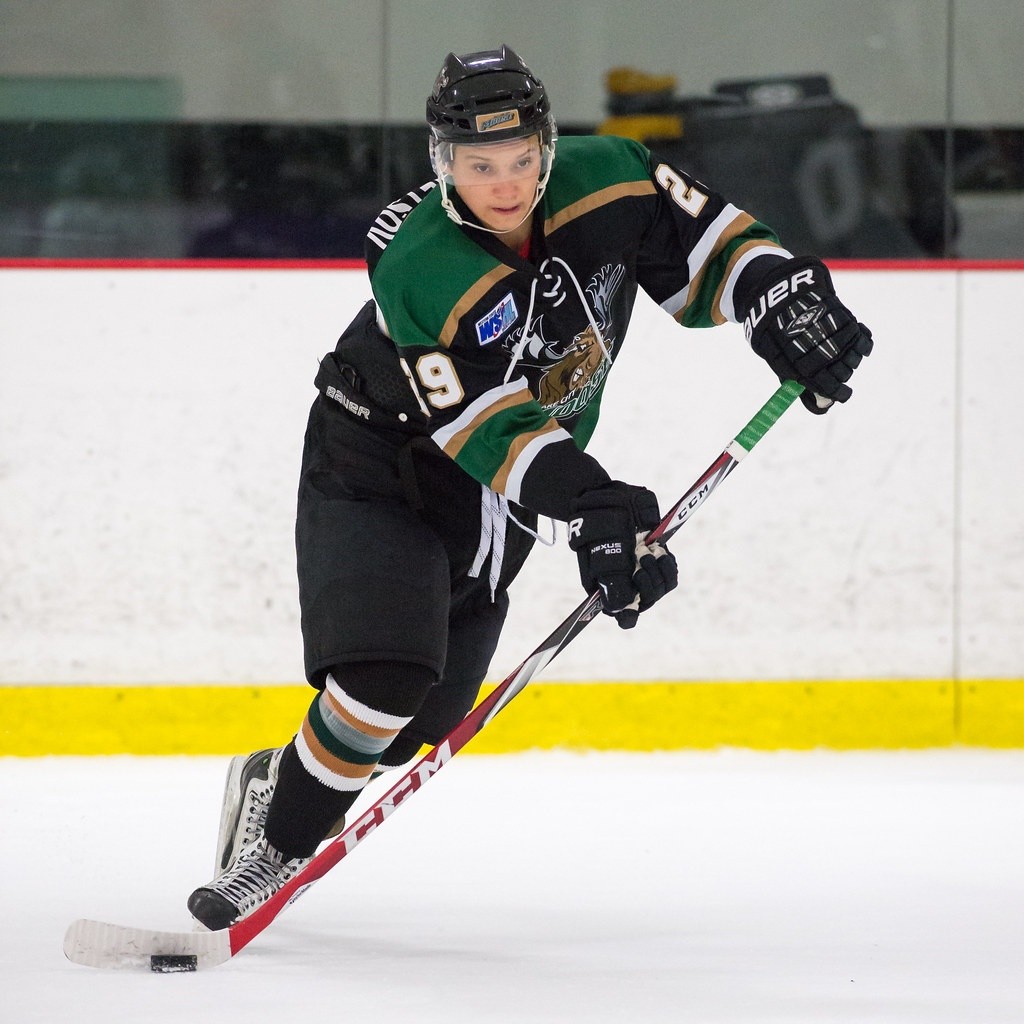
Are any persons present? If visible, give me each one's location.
[186,44,877,938]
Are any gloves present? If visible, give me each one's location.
[567,481,680,630]
[734,255,874,415]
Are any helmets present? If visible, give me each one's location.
[427,44,552,155]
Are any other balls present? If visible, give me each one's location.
[151,952,200,975]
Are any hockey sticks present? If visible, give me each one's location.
[51,379,805,983]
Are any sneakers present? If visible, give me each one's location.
[186,830,314,935]
[213,748,283,878]
